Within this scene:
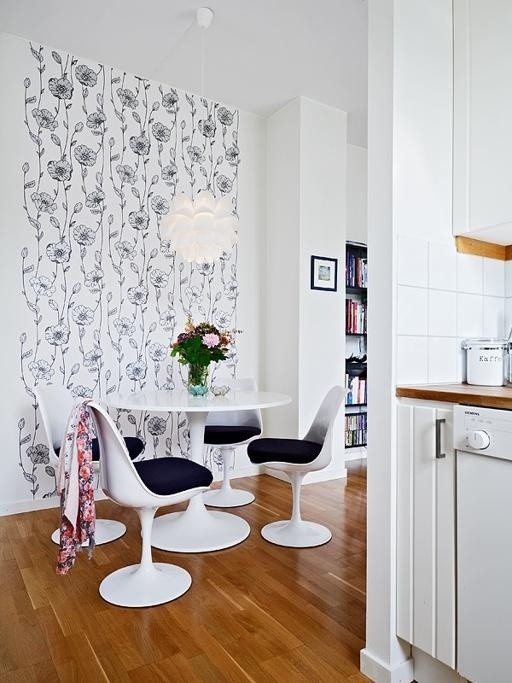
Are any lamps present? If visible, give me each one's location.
[159,7,240,267]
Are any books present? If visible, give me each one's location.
[346,250,367,288]
[345,414,367,447]
[346,299,367,334]
[345,374,367,405]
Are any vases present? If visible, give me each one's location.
[187,364,209,397]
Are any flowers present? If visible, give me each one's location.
[170,321,243,386]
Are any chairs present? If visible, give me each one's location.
[86,402,214,609]
[187,378,263,508]
[247,384,343,548]
[32,384,145,547]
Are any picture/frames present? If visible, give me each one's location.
[311,255,338,292]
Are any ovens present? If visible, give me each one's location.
[451,405,512,683]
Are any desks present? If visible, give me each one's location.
[101,392,293,554]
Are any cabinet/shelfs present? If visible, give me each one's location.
[453,0,512,247]
[396,396,458,671]
[346,286,367,448]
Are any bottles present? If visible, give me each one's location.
[463,336,508,387]
